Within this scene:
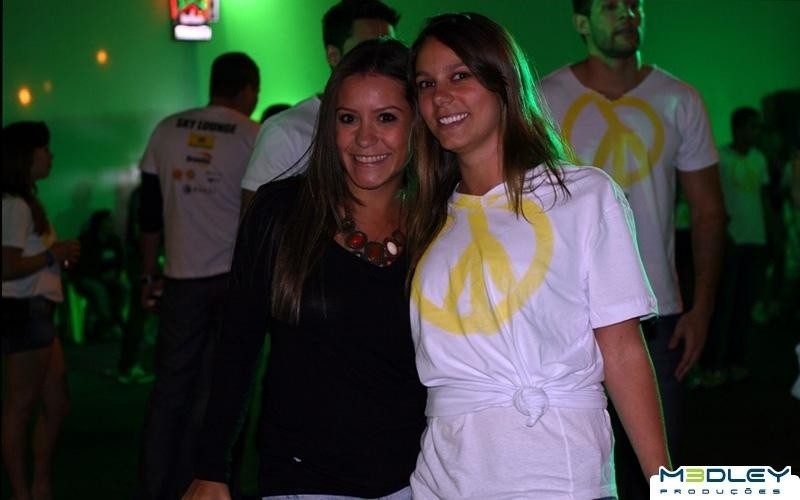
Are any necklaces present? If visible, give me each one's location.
[331,209,408,266]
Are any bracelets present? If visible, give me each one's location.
[39,247,51,265]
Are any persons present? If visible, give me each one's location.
[2,53,293,496]
[183,38,457,498]
[237,0,401,218]
[384,13,676,498]
[527,2,721,474]
[713,83,798,474]
